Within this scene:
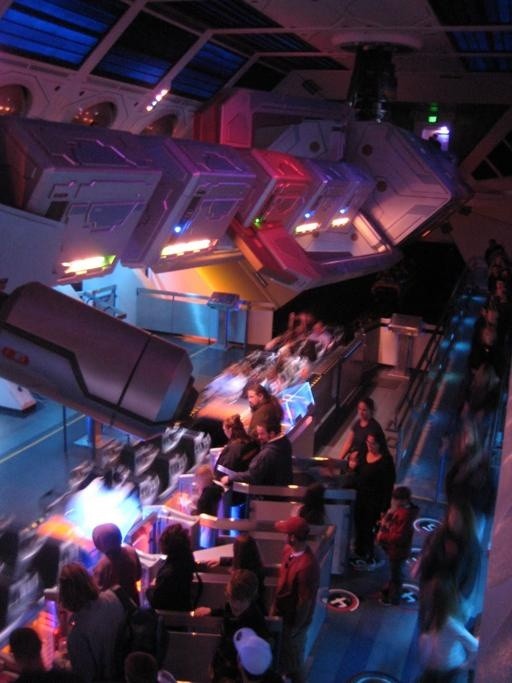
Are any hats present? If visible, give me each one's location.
[274,516,310,537]
[233,628,273,675]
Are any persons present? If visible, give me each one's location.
[91,522,142,607]
[342,396,417,605]
[147,385,329,682]
[414,443,497,682]
[451,238,512,464]
[9,628,83,683]
[123,650,177,683]
[205,307,336,404]
[58,562,137,683]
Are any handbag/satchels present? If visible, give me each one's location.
[110,583,169,682]
